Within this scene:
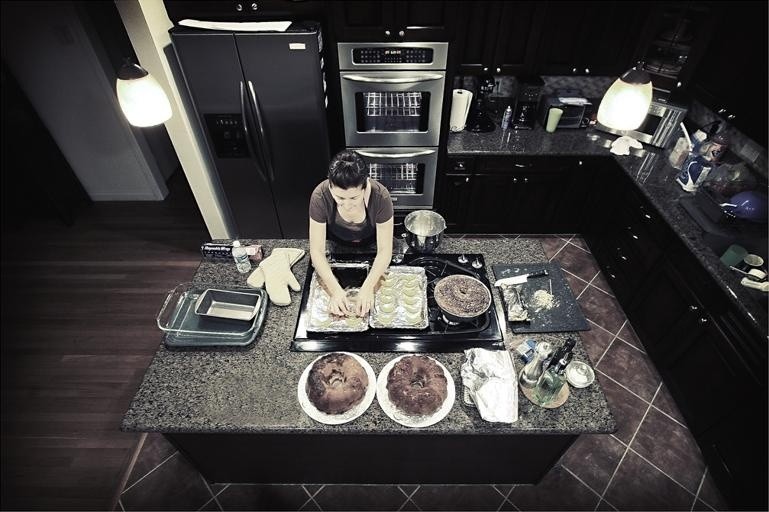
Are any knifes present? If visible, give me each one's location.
[493,270,549,287]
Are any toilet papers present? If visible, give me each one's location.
[449,89,474,131]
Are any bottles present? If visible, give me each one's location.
[500,106,512,132]
[702,114,736,163]
[231,239,251,273]
[520,338,576,405]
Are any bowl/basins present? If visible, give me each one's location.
[404,209,445,252]
[434,273,493,323]
[565,360,595,388]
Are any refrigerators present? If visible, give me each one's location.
[167,23,337,240]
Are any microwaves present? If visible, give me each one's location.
[593,97,689,150]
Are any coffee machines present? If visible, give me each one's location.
[509,74,546,131]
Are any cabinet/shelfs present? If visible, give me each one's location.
[627,244,769,484]
[585,161,670,308]
[560,154,608,237]
[443,156,561,232]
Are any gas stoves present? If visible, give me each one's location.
[289,252,506,353]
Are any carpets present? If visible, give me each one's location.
[114,235,721,508]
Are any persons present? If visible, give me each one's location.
[308,150,395,320]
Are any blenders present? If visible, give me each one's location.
[457,71,496,134]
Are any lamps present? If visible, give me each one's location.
[596,57,653,131]
[111,52,175,129]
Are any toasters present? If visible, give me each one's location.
[537,92,593,130]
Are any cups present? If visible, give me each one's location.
[545,108,564,133]
[719,244,748,270]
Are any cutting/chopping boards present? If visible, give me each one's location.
[491,261,592,334]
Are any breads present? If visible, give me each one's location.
[306,353,367,414]
[386,355,446,417]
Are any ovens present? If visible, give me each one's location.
[345,147,440,212]
[337,40,450,147]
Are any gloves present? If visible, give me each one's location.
[245,247,306,307]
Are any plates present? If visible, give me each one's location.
[376,354,456,428]
[297,351,377,425]
[743,254,765,266]
[518,363,569,409]
